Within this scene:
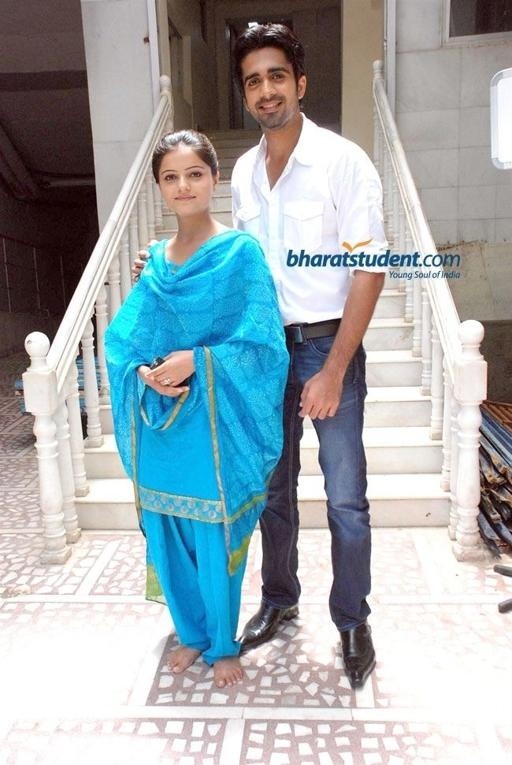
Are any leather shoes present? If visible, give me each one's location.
[236,597,301,651]
[339,623,377,692]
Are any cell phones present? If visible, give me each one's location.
[149,358,189,388]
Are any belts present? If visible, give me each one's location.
[283,318,342,345]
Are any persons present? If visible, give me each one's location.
[132,20,388,690]
[101,131,289,688]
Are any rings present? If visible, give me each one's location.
[164,379,170,385]
